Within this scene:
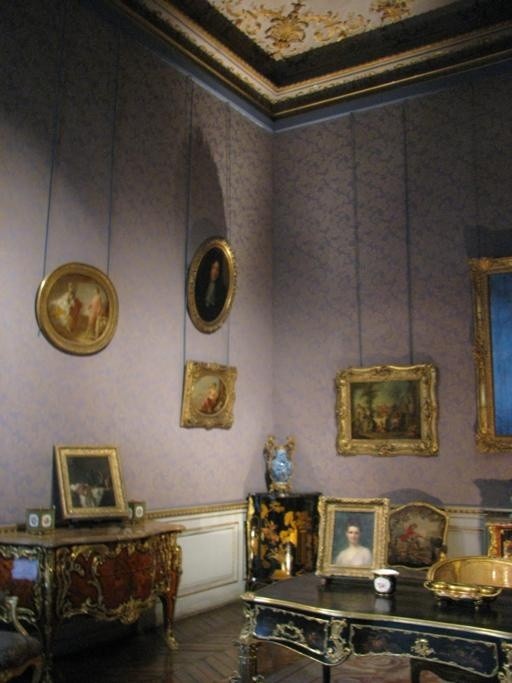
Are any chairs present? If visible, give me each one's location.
[0,594,52,683]
[387,503,448,570]
[410,555,512,683]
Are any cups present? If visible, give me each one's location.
[373,568,400,597]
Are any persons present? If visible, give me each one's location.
[198,258,224,318]
[84,286,103,338]
[334,515,372,565]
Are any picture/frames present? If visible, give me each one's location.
[180,360,238,430]
[34,262,118,355]
[185,236,236,334]
[468,255,512,456]
[316,496,390,584]
[54,443,129,519]
[335,362,439,458]
[486,522,512,560]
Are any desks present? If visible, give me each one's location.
[245,491,322,591]
[0,521,186,658]
[229,571,512,683]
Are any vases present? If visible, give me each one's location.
[267,435,295,494]
